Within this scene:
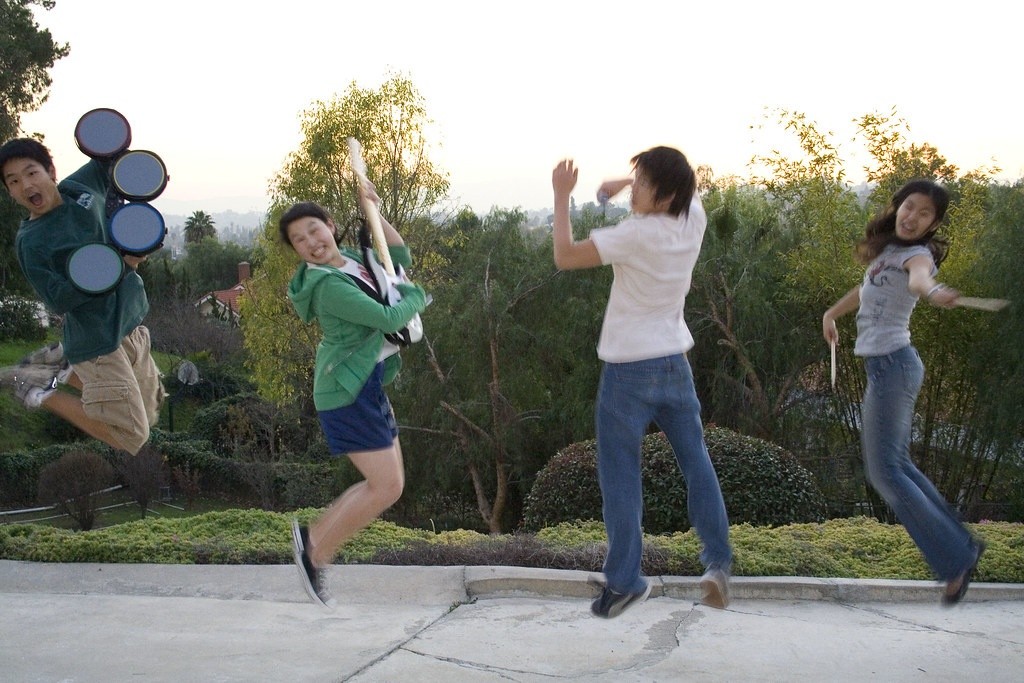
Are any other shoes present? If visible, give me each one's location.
[944,541,985,602]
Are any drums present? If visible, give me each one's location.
[73,108,131,160]
[112,150,167,202]
[65,241,125,296]
[108,202,165,253]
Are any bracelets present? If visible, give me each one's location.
[927,282,952,303]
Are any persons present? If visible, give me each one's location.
[552,145,733,618]
[278,173,426,614]
[823,183,987,607]
[0,137,168,457]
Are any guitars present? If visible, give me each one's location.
[346,136,423,346]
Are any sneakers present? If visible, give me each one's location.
[293,525,312,552]
[587,578,652,619]
[21,341,64,363]
[296,553,333,612]
[0,364,57,402]
[698,569,730,609]
